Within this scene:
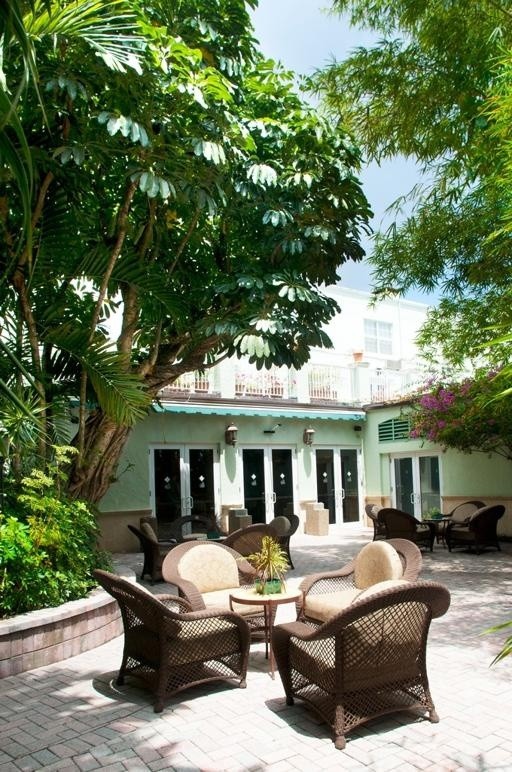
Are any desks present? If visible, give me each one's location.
[224,587,309,681]
[422,517,453,549]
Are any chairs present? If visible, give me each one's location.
[364,502,387,542]
[375,506,437,553]
[125,510,300,585]
[91,566,254,714]
[269,576,455,751]
[435,499,487,546]
[158,538,278,642]
[444,503,506,556]
[294,537,425,623]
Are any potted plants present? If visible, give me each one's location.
[233,535,293,597]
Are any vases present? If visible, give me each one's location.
[183,380,286,399]
[352,351,363,362]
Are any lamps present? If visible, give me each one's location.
[225,419,238,448]
[302,422,316,450]
